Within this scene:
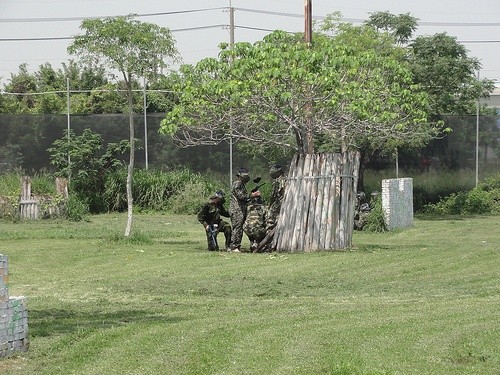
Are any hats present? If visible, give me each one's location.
[235,172,251,182]
[209,189,226,200]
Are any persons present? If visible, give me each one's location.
[198,188,232,252]
[265,164,287,234]
[230,172,260,252]
[243,195,267,251]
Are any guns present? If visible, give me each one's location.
[205,223,220,251]
[251,182,266,193]
